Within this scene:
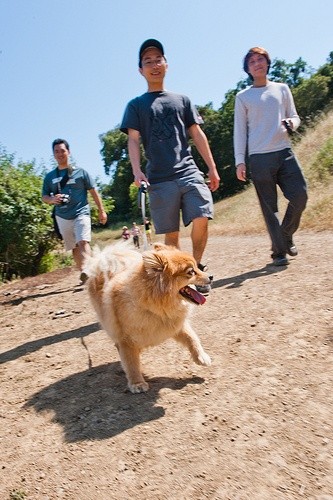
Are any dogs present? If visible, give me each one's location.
[80,237,213,394]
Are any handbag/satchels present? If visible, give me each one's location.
[50,165,74,240]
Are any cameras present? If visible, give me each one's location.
[62,194,71,203]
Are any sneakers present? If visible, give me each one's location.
[273,255,288,266]
[287,245,298,256]
[195,264,211,296]
[80,271,90,288]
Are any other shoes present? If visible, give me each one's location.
[138,246,141,249]
[150,243,153,245]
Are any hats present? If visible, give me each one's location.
[138,39,164,61]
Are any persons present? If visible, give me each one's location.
[120,38,221,296]
[121,226,130,241]
[142,217,152,245]
[41,138,107,283]
[234,46,308,265]
[131,222,142,249]
